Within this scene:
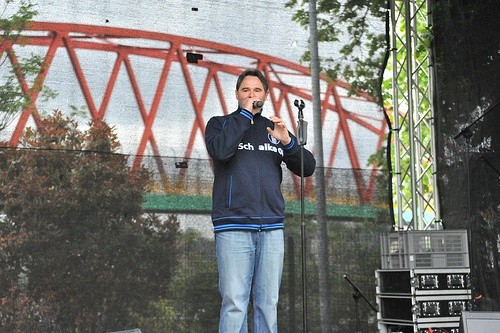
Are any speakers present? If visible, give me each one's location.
[459,311,500,333]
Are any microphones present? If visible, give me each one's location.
[253,101,262,108]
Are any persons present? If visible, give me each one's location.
[203,68,318,333]
